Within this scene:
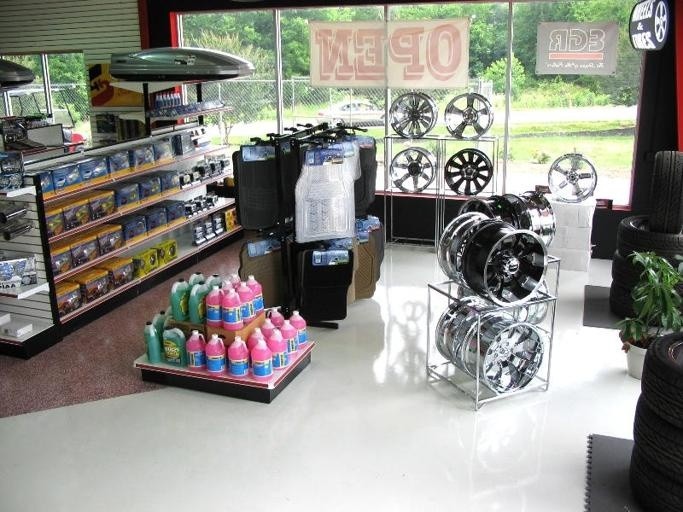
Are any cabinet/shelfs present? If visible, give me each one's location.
[0,79,244,361]
[133,309,312,403]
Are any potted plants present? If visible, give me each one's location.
[614,249,683,380]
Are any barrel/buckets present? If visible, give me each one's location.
[261,318,275,337]
[185,330,206,371]
[205,333,227,377]
[189,271,205,290]
[248,327,266,356]
[251,339,273,380]
[205,285,224,327]
[267,329,290,370]
[246,274,264,315]
[236,282,256,322]
[153,311,166,336]
[143,321,161,364]
[230,273,241,290]
[169,278,189,320]
[265,308,284,328]
[280,319,299,359]
[228,336,249,378]
[290,311,308,350]
[222,280,234,295]
[188,280,209,324]
[205,273,223,292]
[222,289,244,330]
[162,326,186,366]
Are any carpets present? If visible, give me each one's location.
[0,232,265,418]
